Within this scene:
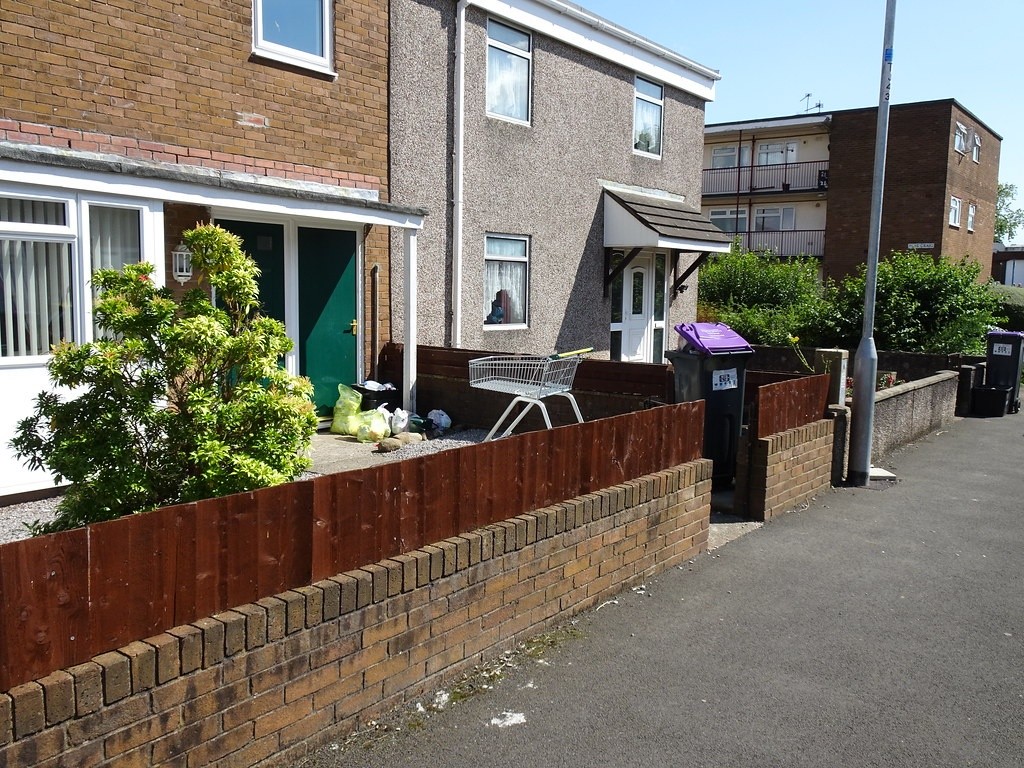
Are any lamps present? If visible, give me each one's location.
[172,240,194,286]
[676,285,689,294]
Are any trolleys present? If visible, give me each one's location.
[469,346,593,441]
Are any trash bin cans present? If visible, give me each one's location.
[975,385,1013,417]
[663,322,756,491]
[984,332,1024,413]
[351,383,400,414]
